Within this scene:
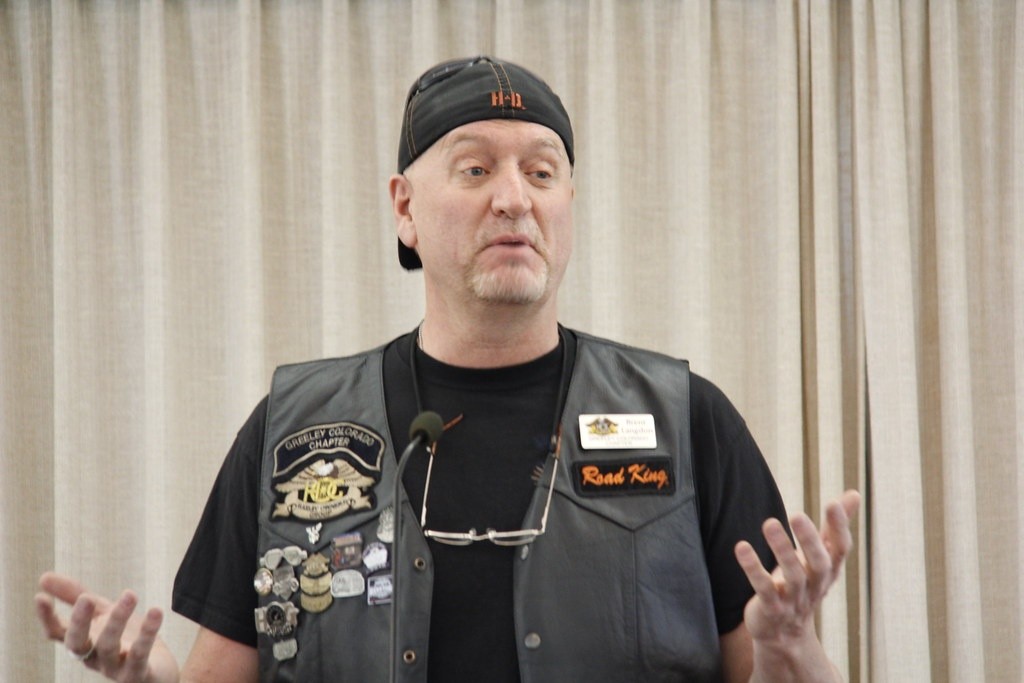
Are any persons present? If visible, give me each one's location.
[36,55,861,683]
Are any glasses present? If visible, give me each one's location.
[407,56,493,111]
[420,411,562,547]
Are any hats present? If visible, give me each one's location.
[396,55,576,272]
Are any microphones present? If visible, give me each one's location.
[387,412,444,683]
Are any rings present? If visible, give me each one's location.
[71,644,94,661]
